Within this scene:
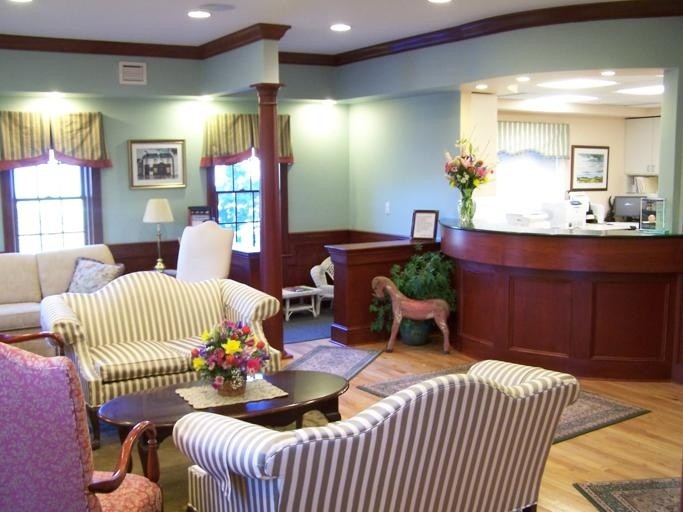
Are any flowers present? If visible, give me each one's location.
[441,135,499,212]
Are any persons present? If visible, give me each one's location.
[136,157,172,178]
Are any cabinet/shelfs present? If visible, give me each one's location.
[622,114,661,178]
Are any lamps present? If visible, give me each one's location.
[141,196,177,270]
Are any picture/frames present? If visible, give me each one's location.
[126,137,187,192]
[409,210,440,244]
[570,145,610,191]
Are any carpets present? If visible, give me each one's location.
[356,358,652,453]
[572,476,682,511]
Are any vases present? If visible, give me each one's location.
[456,188,477,231]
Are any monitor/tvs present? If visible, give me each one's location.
[615,195,647,222]
